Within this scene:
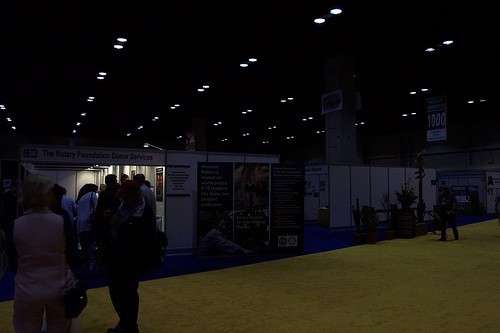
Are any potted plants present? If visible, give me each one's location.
[361,205,380,244]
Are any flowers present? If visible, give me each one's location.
[396,174,418,204]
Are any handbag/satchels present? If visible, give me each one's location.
[89,212,96,223]
[155,231,168,248]
[63,282,88,319]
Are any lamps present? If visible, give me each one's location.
[143,142,163,150]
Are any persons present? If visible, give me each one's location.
[494,195,500,222]
[0,182,24,274]
[104,181,156,333]
[47,183,78,253]
[212,219,254,259]
[74,173,156,274]
[438,186,459,240]
[197,220,218,254]
[13,176,72,333]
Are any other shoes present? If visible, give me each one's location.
[455,237,459,240]
[108,326,139,333]
[439,236,446,241]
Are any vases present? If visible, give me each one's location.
[401,203,413,210]
[383,230,395,241]
